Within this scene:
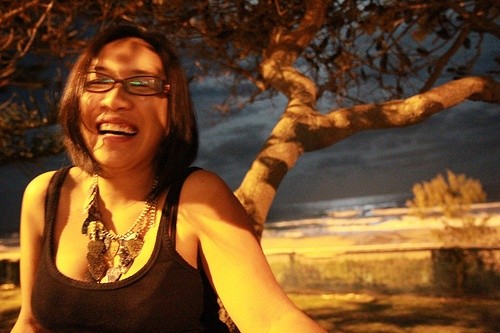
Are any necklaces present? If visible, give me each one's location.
[82,168,160,282]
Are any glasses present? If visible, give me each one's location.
[80,71,171,95]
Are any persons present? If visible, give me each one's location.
[9,24,328,333]
